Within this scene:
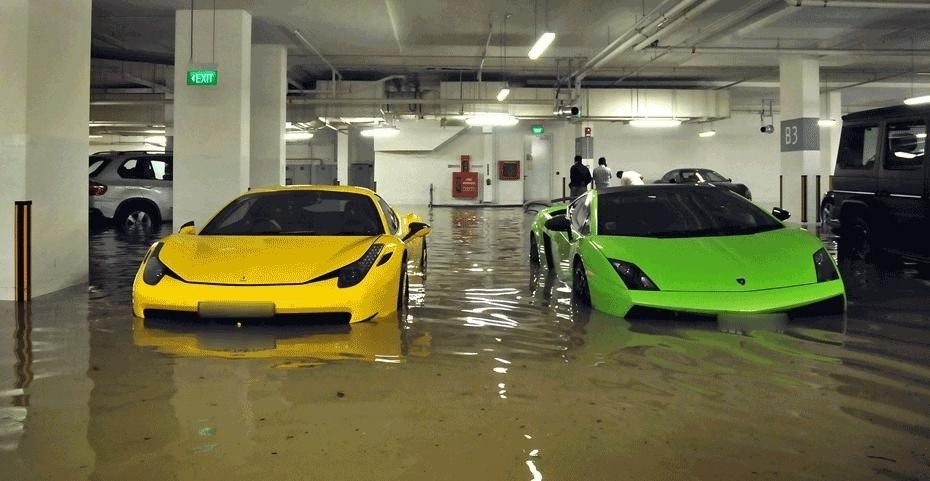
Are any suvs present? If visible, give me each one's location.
[88,145,173,239]
[825,99,929,303]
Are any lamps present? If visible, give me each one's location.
[527,0,557,63]
[903,36,930,106]
[496,14,513,104]
[816,66,838,128]
[696,120,717,139]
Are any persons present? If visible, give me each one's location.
[568,155,591,202]
[616,170,644,186]
[592,157,612,190]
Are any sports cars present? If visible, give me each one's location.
[648,166,755,202]
[125,180,428,326]
[525,180,855,322]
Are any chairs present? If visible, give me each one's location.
[891,131,920,165]
